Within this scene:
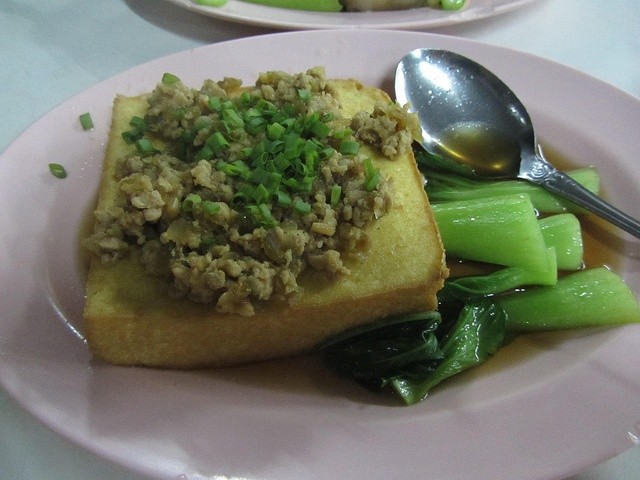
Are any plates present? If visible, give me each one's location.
[2,30,639,474]
[162,0,548,31]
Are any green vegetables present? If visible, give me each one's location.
[315,152,632,408]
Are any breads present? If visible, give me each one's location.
[83,79,450,370]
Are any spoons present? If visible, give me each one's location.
[395,47,639,264]
